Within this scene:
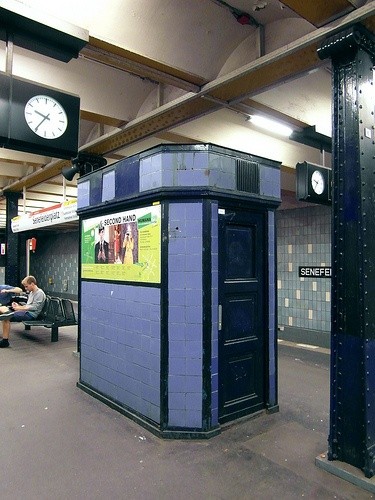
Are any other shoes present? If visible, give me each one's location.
[0,338,10,349]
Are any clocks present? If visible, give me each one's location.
[311,170,325,195]
[23,94,68,140]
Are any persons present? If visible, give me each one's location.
[0,284,23,314]
[0,275,47,348]
[94,221,135,265]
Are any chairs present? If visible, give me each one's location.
[22,294,79,342]
